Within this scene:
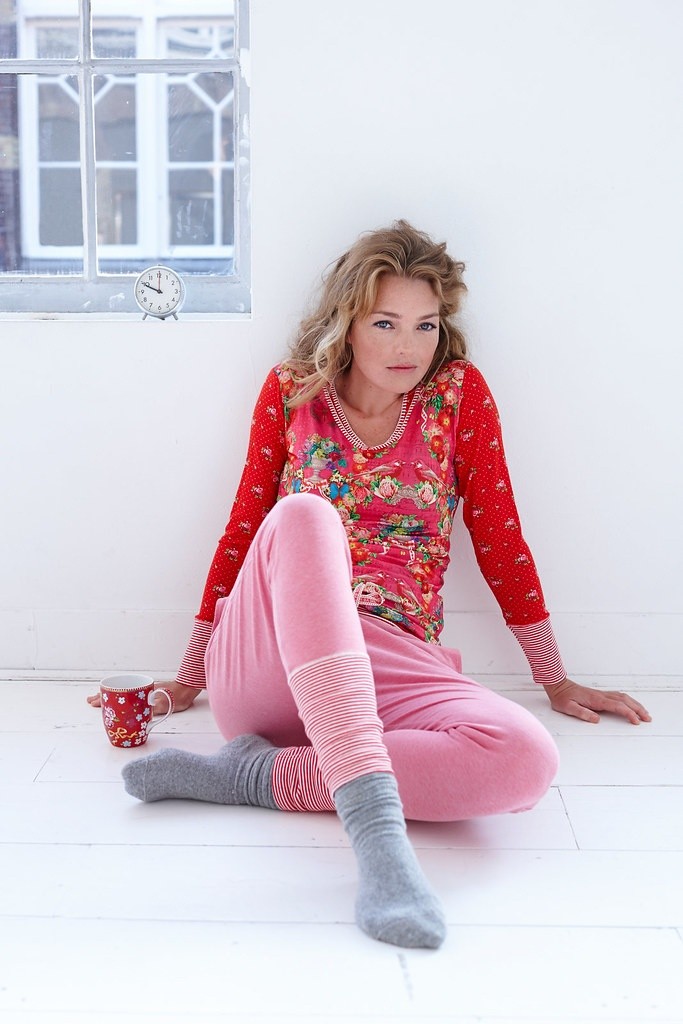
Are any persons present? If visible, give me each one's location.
[87,220,651,950]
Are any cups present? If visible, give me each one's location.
[99,674,175,749]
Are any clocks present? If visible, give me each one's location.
[135,263,185,321]
